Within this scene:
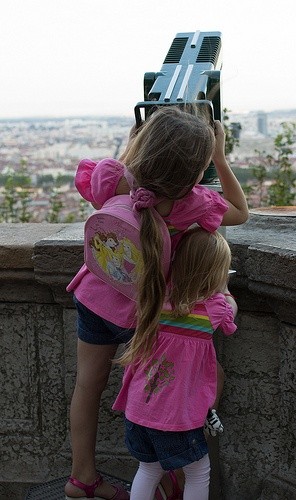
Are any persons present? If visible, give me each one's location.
[61,103,249,500]
[111,224,238,499]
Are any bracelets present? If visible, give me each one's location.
[223,294,235,298]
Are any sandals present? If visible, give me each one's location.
[65,473,132,500]
[153,471,181,500]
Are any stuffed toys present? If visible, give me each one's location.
[204,407,225,437]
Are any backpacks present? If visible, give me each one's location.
[83,193,172,304]
